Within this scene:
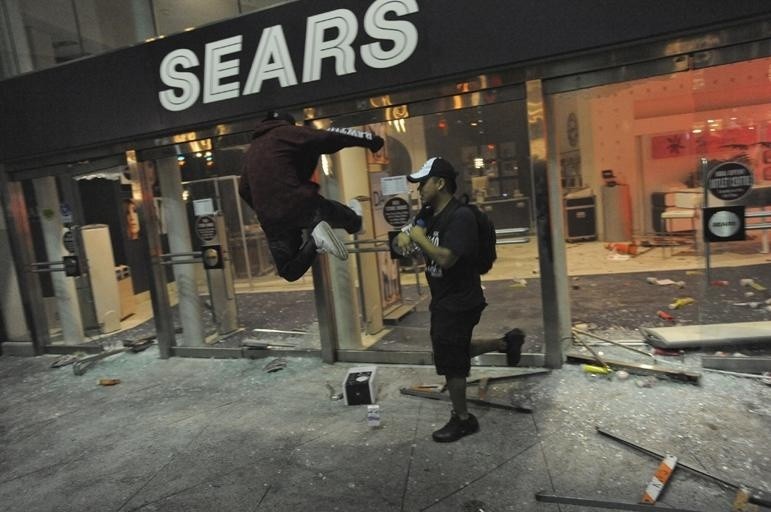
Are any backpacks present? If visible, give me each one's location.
[445,193,496,274]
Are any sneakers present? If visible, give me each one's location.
[310,220,349,261]
[349,199,366,234]
[432,414,478,443]
[498,328,525,367]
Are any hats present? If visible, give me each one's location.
[407,157,459,183]
[261,111,295,124]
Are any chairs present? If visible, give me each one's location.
[660,193,698,258]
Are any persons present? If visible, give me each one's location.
[237,106,387,281]
[391,155,526,444]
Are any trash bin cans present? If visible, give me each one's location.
[563,193,598,243]
